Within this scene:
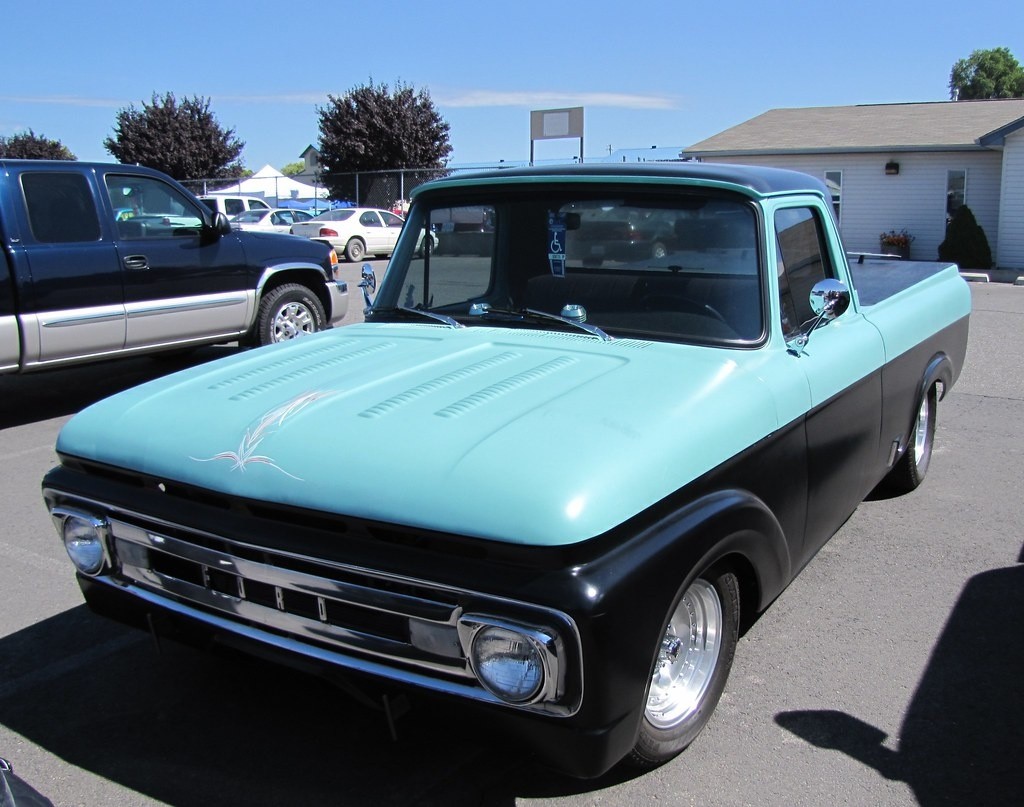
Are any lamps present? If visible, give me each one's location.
[885,162,899,176]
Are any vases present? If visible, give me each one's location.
[881,245,910,261]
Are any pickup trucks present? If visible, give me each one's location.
[1,156,343,389]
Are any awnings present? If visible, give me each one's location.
[304,199,336,210]
[332,198,356,209]
[278,199,312,210]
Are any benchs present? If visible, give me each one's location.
[516,270,773,343]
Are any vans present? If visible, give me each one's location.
[193,193,274,223]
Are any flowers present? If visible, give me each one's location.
[879,227,916,247]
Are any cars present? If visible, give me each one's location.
[39,165,970,775]
